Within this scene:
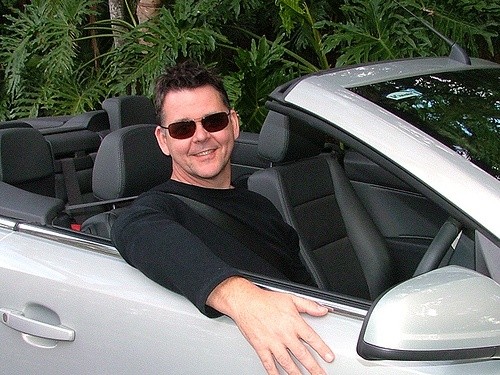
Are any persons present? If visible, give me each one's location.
[111,61,335,375]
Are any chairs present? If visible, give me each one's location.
[0,95,396,302]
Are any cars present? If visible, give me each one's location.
[0,42,500,375]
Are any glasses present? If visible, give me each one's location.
[159,111,231,139]
